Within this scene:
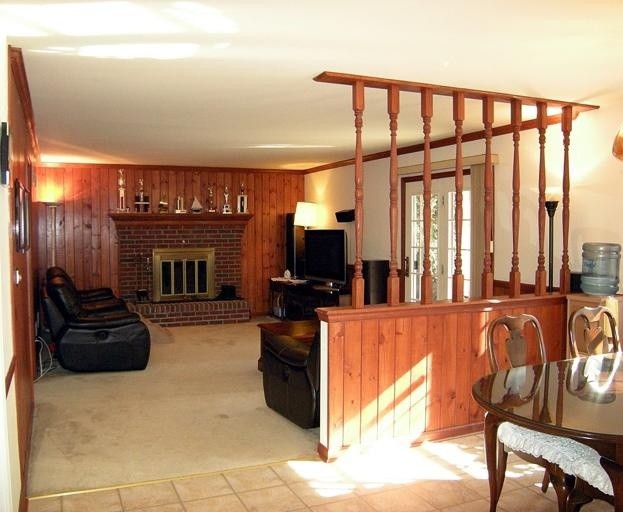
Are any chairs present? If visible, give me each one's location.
[482,303,621,494]
[260,326,319,429]
[40,267,150,373]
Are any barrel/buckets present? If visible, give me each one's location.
[578,360,617,404]
[580,243,621,295]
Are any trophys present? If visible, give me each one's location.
[115,167,248,215]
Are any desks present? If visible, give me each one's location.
[471,351,622,510]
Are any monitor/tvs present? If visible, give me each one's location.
[304,230,346,291]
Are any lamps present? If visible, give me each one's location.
[542,195,563,290]
[42,201,63,266]
[292,199,317,230]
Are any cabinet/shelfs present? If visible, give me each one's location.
[283,280,352,320]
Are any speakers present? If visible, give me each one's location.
[362,260,390,305]
[336,210,354,222]
[285,213,304,280]
